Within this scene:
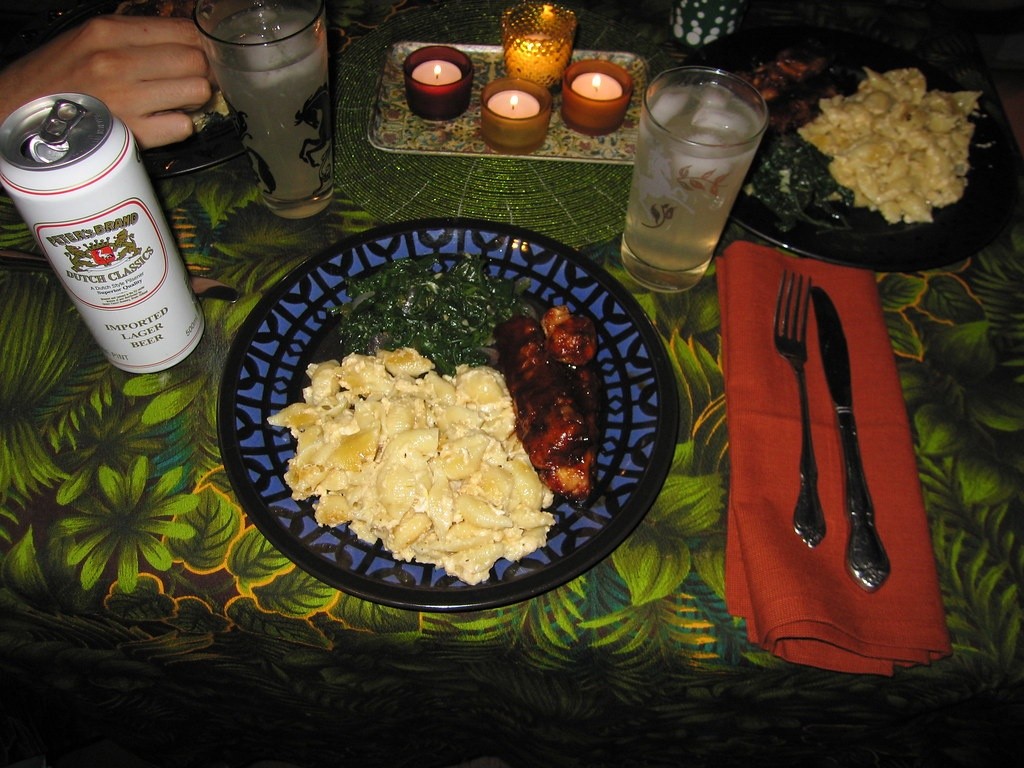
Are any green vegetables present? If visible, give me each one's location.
[329,250,541,376]
[738,130,854,233]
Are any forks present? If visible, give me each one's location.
[770,269,827,548]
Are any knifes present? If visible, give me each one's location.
[809,285,891,592]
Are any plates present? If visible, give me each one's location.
[684,29,1023,273]
[216,217,679,612]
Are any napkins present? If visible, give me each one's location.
[701,240,953,677]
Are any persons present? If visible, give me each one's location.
[0,15,222,154]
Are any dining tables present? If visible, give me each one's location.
[0,0,1022,767]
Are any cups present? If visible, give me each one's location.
[620,66,770,292]
[192,1,335,218]
[501,0,578,93]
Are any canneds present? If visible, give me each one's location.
[0,91,205,374]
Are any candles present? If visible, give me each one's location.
[479,77,553,156]
[402,46,473,120]
[501,1,577,89]
[561,58,633,136]
